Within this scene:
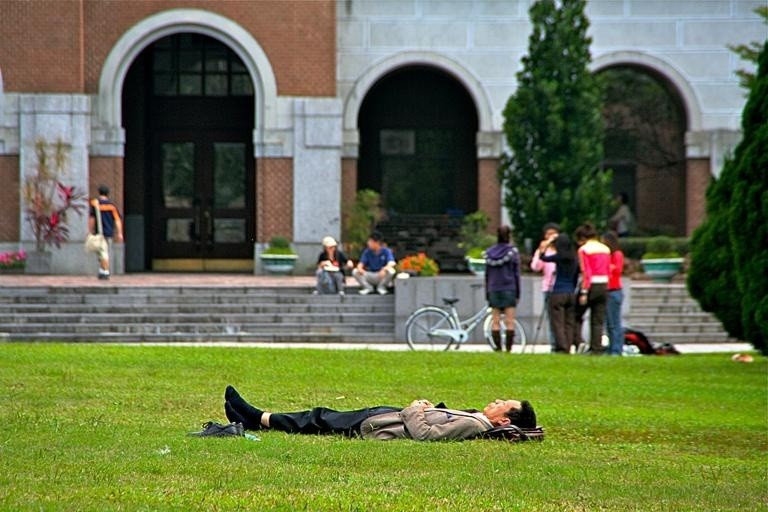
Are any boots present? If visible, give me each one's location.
[492,330,501,351]
[506,330,515,350]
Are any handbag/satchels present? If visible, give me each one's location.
[474,426,544,443]
[85,234,107,254]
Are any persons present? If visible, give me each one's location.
[352,233,397,295]
[481,226,520,353]
[531,222,628,355]
[607,192,632,237]
[311,238,354,297]
[88,185,123,280]
[224,372,537,445]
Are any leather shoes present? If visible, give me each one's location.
[187,421,245,437]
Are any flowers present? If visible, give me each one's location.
[398,253,439,276]
[0,250,27,267]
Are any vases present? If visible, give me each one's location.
[28,250,88,273]
[0,268,24,274]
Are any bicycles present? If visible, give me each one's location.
[401,281,526,354]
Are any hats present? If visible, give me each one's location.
[324,236,337,247]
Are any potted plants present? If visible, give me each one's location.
[457,210,499,275]
[261,236,298,275]
[342,189,386,275]
[24,140,91,249]
[639,235,684,286]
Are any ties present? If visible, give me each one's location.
[435,402,479,412]
